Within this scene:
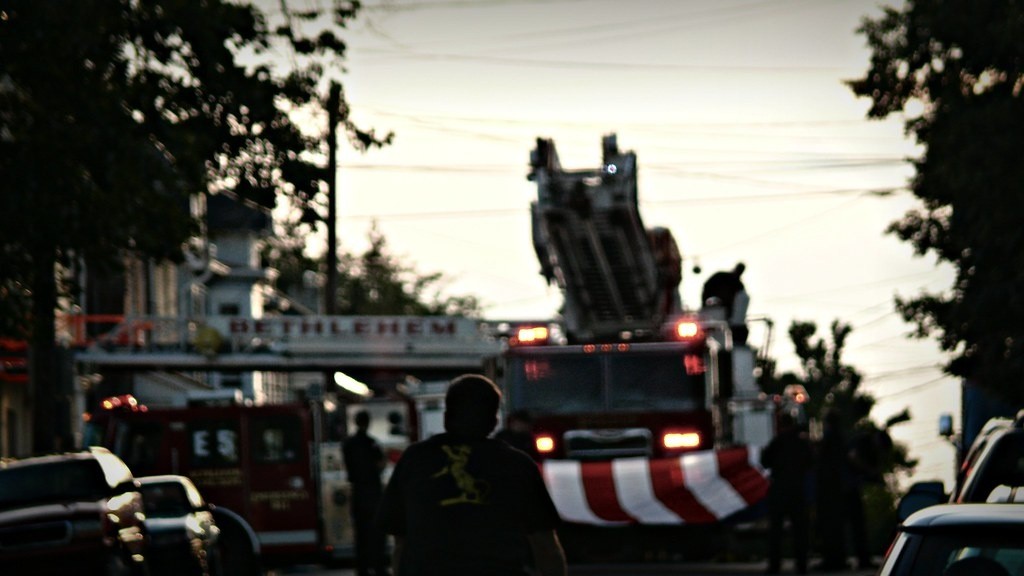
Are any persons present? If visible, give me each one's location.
[699,263,745,319]
[760,415,822,576]
[817,410,882,575]
[343,408,393,575]
[353,372,570,576]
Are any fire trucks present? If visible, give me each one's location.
[490,134,792,562]
[103,378,469,542]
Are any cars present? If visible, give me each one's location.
[874,408,1024,574]
[0,447,273,574]
